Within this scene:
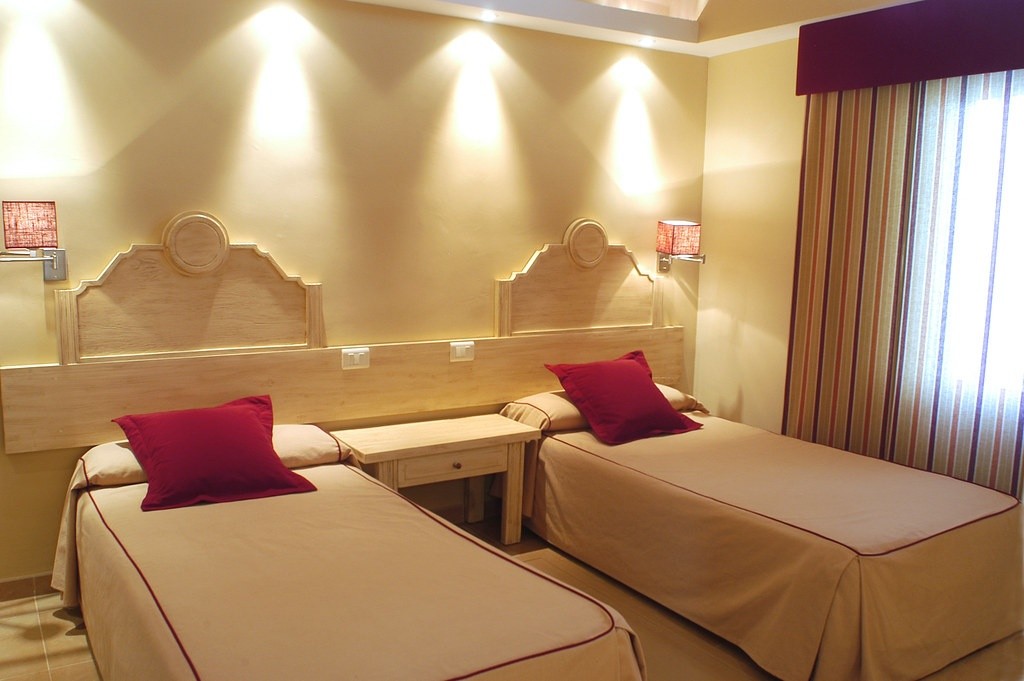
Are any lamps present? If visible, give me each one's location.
[656,219,706,273]
[0,200,69,282]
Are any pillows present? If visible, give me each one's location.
[543,350,704,447]
[489,382,710,518]
[110,394,317,511]
[49,422,360,611]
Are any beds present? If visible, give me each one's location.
[77,463,650,681]
[520,407,1023,680]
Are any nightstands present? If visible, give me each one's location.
[329,413,541,546]
